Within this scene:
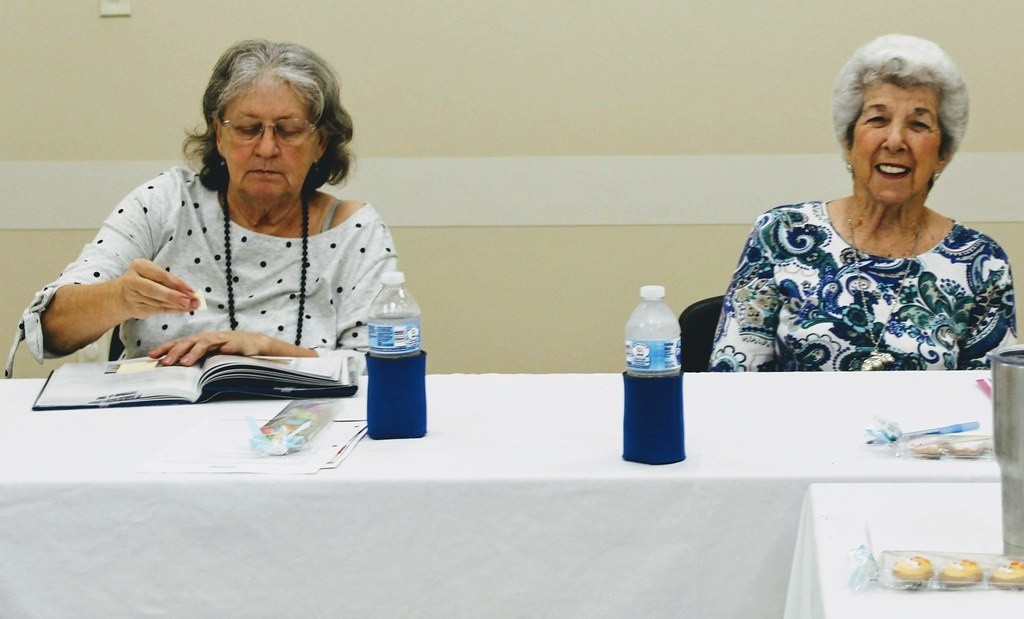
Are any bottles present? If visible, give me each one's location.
[367,271,422,358]
[625,285,682,378]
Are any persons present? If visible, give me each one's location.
[4,40,397,380]
[709,34,1018,373]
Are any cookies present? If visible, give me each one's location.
[891,556,1024,590]
[908,436,984,455]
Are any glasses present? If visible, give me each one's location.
[221,117,316,145]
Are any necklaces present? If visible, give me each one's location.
[223,191,308,347]
[845,197,922,371]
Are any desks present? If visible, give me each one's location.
[783,482,1024,619]
[0,371,1000,619]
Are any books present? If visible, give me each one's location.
[33,349,358,412]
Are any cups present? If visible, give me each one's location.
[623,371,685,464]
[364,350,427,440]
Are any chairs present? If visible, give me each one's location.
[679,295,724,372]
